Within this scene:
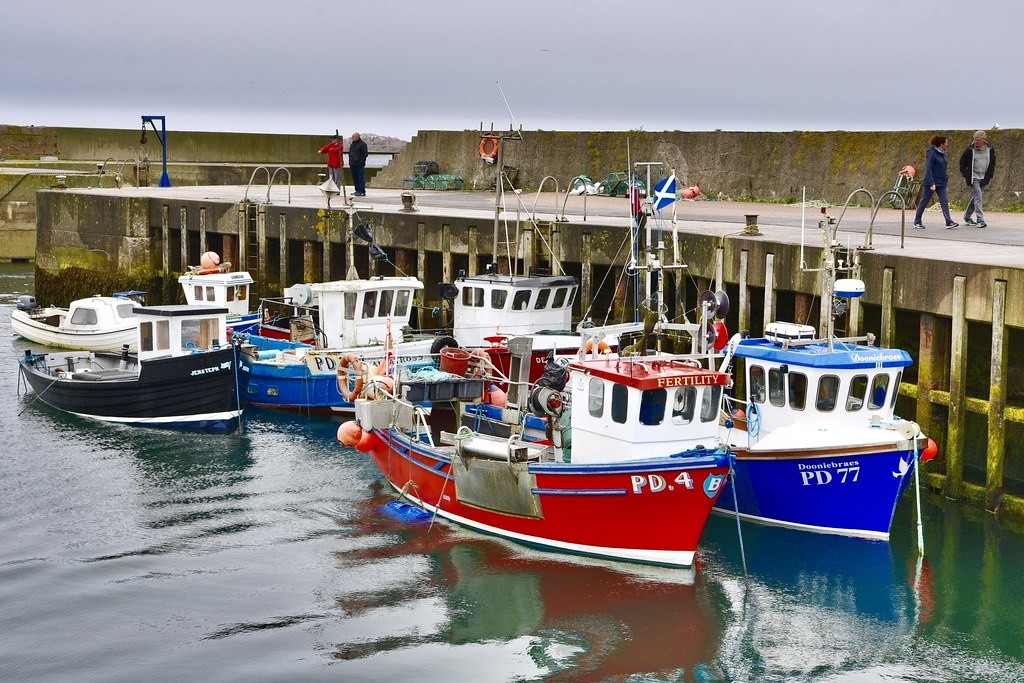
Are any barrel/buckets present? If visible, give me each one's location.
[439,345,471,376]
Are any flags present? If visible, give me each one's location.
[653,173,677,210]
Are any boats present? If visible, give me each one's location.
[18,305,244,435]
[112,252,315,351]
[232,157,928,570]
[10,292,143,355]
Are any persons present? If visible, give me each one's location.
[913,134,960,229]
[958,130,996,228]
[317,135,344,195]
[348,133,368,196]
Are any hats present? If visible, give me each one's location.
[330,135,336,139]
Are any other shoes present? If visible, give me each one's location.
[352,192,365,196]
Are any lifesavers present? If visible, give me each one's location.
[480,135,497,159]
[578,341,611,355]
[469,350,492,390]
[197,267,227,275]
[337,354,364,401]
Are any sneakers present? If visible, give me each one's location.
[914,223,925,229]
[946,222,959,228]
[964,218,977,225]
[976,221,987,228]
